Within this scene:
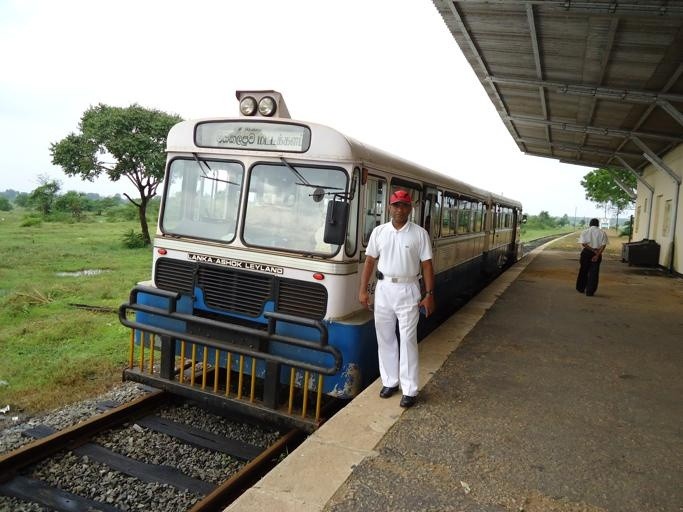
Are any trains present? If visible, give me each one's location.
[130,88,526,403]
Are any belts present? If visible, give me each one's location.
[383,277,418,285]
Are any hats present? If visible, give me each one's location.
[389,190,413,204]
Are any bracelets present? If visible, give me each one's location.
[426,290,433,295]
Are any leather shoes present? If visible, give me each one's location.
[400,395,417,408]
[380,384,399,398]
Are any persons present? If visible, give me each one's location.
[358,191,435,406]
[576,219,608,296]
